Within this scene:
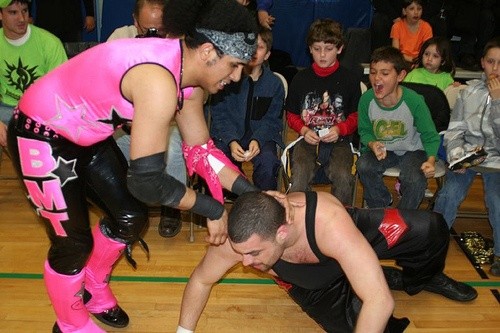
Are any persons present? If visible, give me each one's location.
[0,0,500,333]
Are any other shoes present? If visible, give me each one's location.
[489,257,500,276]
[364,193,393,208]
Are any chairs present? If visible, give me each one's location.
[198,72,288,229]
[309,82,367,208]
[363,83,452,209]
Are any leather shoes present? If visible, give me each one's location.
[424,272,478,302]
[52,321,61,333]
[381,265,410,291]
[158,204,182,237]
[81,288,129,327]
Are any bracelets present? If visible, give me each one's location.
[177,325,194,333]
